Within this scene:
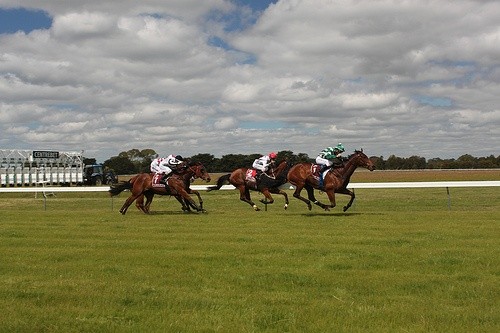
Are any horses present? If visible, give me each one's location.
[106,157,211,216]
[206,152,307,212]
[283,147,375,213]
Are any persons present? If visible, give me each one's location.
[252,153,277,172]
[150,154,183,175]
[316,143,345,179]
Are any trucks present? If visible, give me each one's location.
[0,148,116,188]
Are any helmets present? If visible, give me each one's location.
[269,152,277,159]
[335,143,345,152]
